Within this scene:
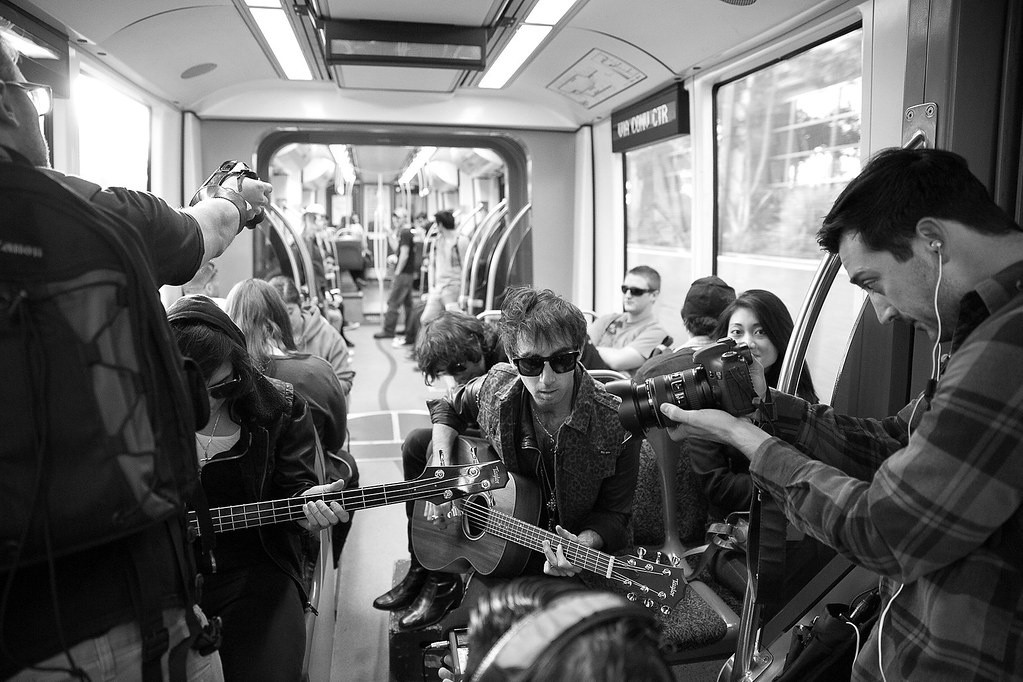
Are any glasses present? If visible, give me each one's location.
[207,368,243,400]
[509,346,581,377]
[621,285,655,296]
[433,358,468,376]
[5,80,54,118]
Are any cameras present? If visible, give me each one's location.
[202,160,266,229]
[605,337,756,445]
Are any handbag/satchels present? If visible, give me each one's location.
[678,510,749,596]
[774,596,883,682]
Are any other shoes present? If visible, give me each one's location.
[343,319,360,330]
[393,336,415,351]
[373,328,395,338]
[356,277,368,286]
[396,330,408,335]
[344,337,355,365]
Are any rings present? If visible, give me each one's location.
[550,565,555,569]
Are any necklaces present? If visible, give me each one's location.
[531,406,559,453]
[195,408,221,464]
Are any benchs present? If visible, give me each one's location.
[584,368,763,665]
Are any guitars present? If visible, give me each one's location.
[410,435,690,616]
[180,457,513,576]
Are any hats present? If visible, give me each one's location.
[684,276,736,332]
[393,207,408,219]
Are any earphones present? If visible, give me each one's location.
[931,239,943,251]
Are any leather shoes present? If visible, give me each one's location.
[373,553,432,611]
[398,570,463,632]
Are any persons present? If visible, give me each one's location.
[0,37,274,682]
[158,204,817,682]
[666,145,1023,682]
